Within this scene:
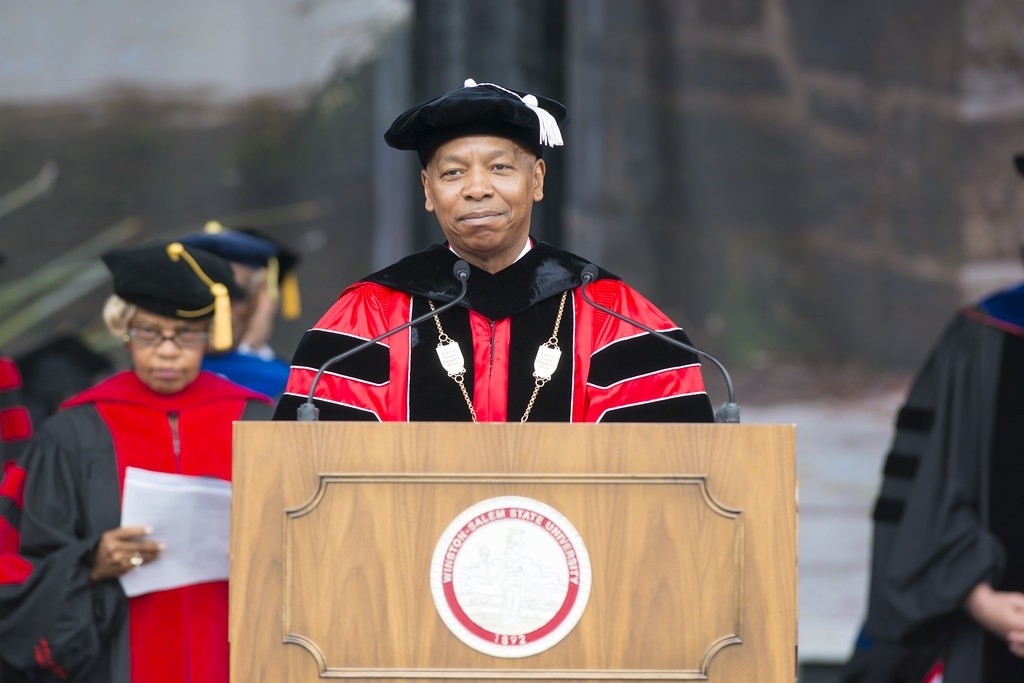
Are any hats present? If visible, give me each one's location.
[99,238,248,351]
[182,223,280,300]
[387,78,567,169]
[237,227,303,321]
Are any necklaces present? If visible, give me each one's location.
[429,292,567,422]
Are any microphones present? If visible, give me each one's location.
[298,260,470,421]
[580,264,741,423]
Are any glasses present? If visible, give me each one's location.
[119,326,212,348]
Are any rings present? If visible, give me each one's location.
[130,551,144,569]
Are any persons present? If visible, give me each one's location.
[273,76,719,423]
[0,225,291,682]
[840,149,1024,683]
